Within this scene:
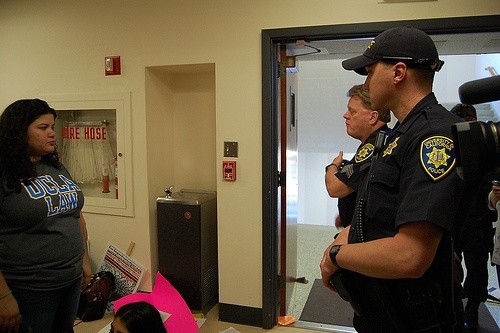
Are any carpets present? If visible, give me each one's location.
[298,279,500,333]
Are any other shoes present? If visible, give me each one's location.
[463,303,479,332]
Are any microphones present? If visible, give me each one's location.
[458,75,500,105]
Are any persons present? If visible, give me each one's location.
[320,25,468,333]
[108,302,168,332]
[326,84,500,333]
[0,99,92,333]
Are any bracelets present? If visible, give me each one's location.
[1,289,12,299]
[325,165,338,171]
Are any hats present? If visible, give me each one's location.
[451,104,476,118]
[342,26,444,76]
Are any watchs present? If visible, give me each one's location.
[329,245,341,268]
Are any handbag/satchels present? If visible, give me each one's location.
[77,271,115,322]
